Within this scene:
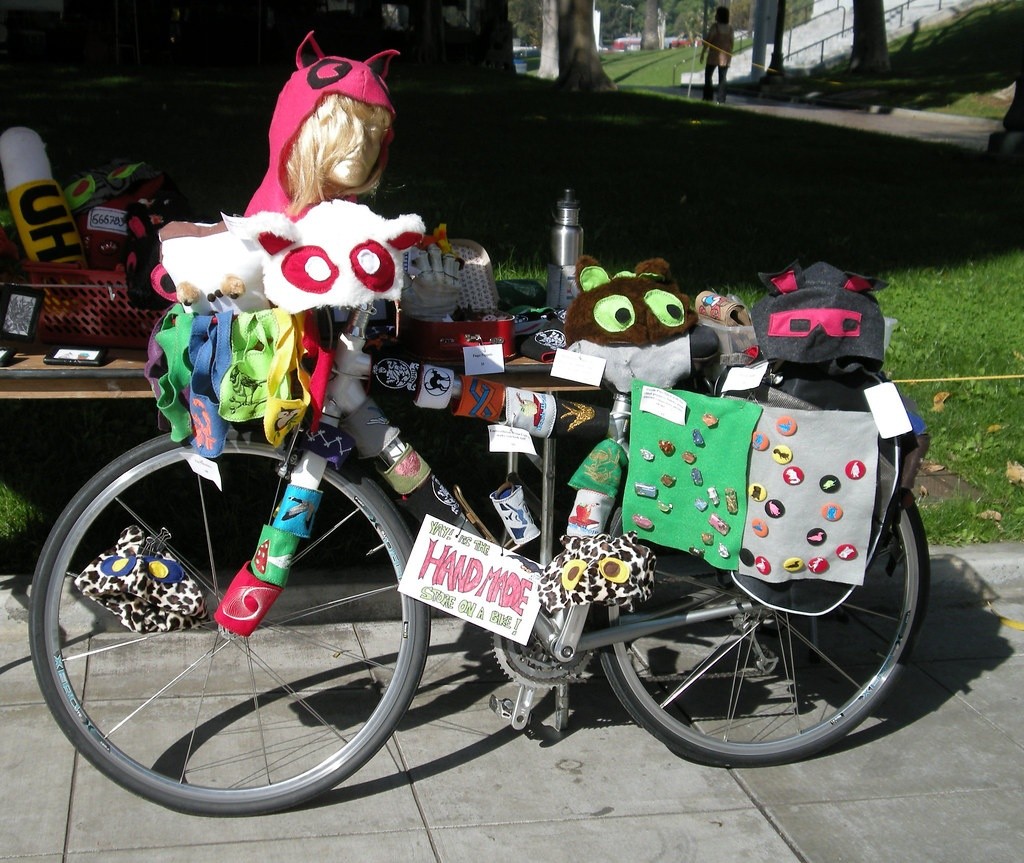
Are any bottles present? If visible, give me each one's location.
[549,192,585,308]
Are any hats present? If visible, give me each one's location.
[564,256,698,348]
[220,199,426,313]
[538,531,657,612]
[752,262,885,372]
[73,526,210,633]
[243,28,402,218]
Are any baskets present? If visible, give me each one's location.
[21,258,166,347]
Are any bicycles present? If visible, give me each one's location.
[29,293,932,820]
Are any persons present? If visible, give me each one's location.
[250,30,403,219]
[699,6,734,104]
